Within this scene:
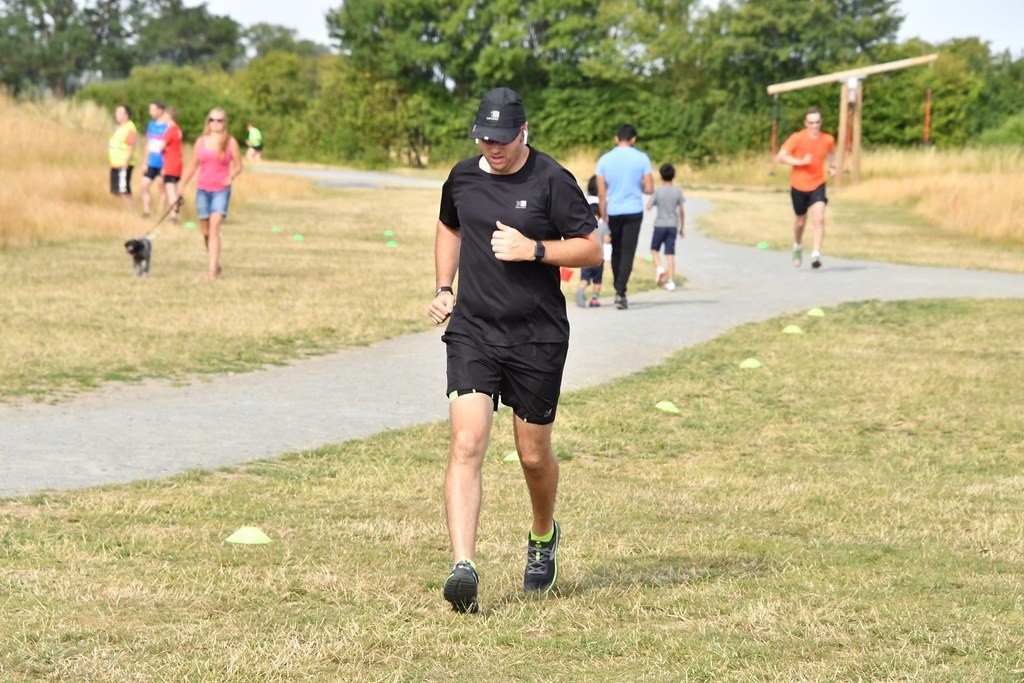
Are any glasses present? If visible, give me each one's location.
[208,118,226,122]
[805,120,822,124]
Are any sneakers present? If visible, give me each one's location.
[523,518,560,594]
[443,560,478,614]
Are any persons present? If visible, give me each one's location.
[429,87,602,614]
[244,124,263,160]
[155,108,182,220]
[177,106,242,277]
[141,102,169,214]
[648,164,685,287]
[108,106,139,208]
[596,125,653,309]
[576,176,601,308]
[779,110,836,267]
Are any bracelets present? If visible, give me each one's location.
[436,287,453,298]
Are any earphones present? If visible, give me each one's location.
[523,130,528,145]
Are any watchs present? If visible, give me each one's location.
[534,241,545,263]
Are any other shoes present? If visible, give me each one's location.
[576,288,585,307]
[811,257,822,269]
[792,248,802,267]
[656,267,665,287]
[665,281,676,291]
[589,299,600,307]
[614,295,628,309]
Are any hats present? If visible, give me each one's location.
[469,86,526,144]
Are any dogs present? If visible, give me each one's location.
[124,228,160,277]
